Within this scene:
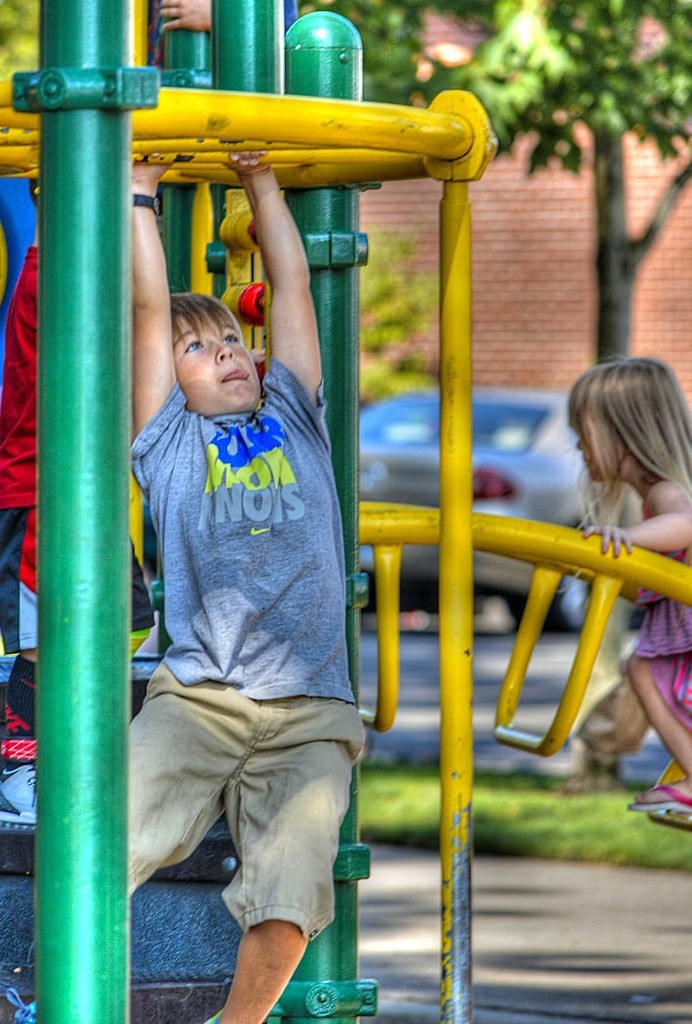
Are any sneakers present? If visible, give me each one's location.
[0,734,37,816]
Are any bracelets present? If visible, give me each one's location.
[133,194,159,215]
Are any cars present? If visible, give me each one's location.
[354,385,596,633]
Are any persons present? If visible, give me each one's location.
[160,0,297,35]
[567,358,692,811]
[7,148,364,1024]
[0,177,154,821]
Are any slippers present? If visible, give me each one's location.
[627,783,692,812]
